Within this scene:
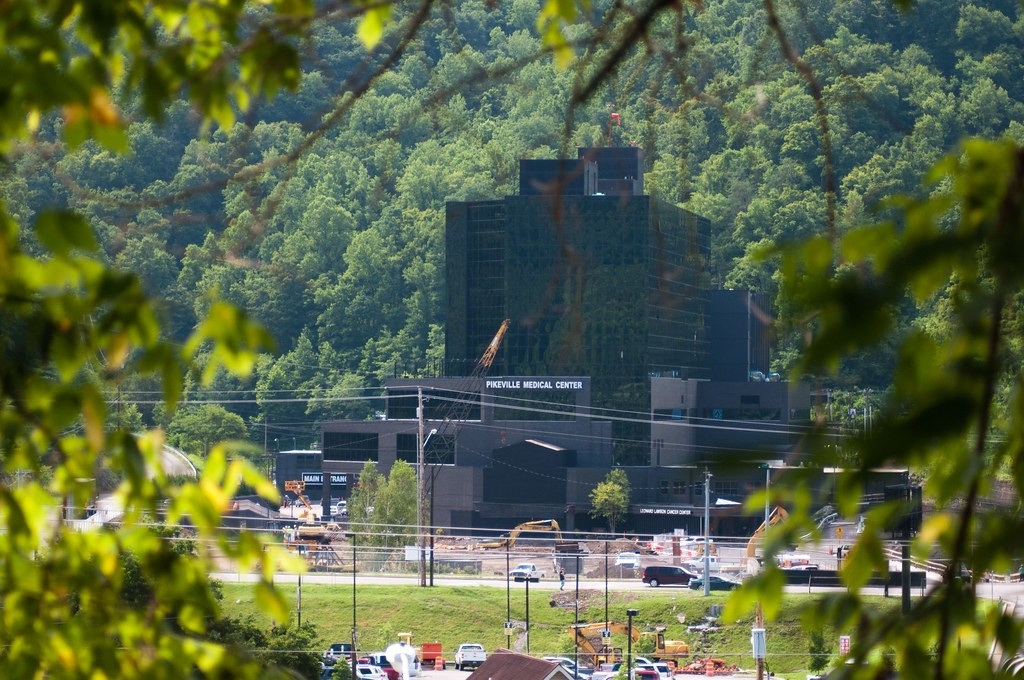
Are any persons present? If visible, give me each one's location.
[1018,565,1024,582]
[984,569,990,583]
[559,568,566,591]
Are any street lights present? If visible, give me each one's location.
[295,544,306,630]
[567,552,589,678]
[625,608,640,680]
[416,428,439,585]
[503,533,512,652]
[515,576,539,655]
[596,535,617,664]
[344,533,356,642]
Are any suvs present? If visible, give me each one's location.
[642,565,699,588]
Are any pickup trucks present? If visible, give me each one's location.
[452,644,488,671]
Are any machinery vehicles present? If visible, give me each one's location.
[283,480,338,551]
[475,518,584,575]
[565,619,691,672]
[745,504,818,573]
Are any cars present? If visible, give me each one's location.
[337,500,346,513]
[509,562,546,583]
[295,495,311,508]
[615,551,641,570]
[687,575,742,592]
[544,656,671,680]
[321,641,422,680]
[685,537,715,552]
